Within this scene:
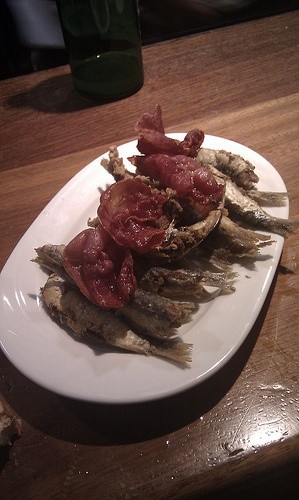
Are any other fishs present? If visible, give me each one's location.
[30,145,299,363]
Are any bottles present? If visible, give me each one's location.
[55,0,145,101]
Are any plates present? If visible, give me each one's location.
[0,132,289,405]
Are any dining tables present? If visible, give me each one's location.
[1,10,299,500]
